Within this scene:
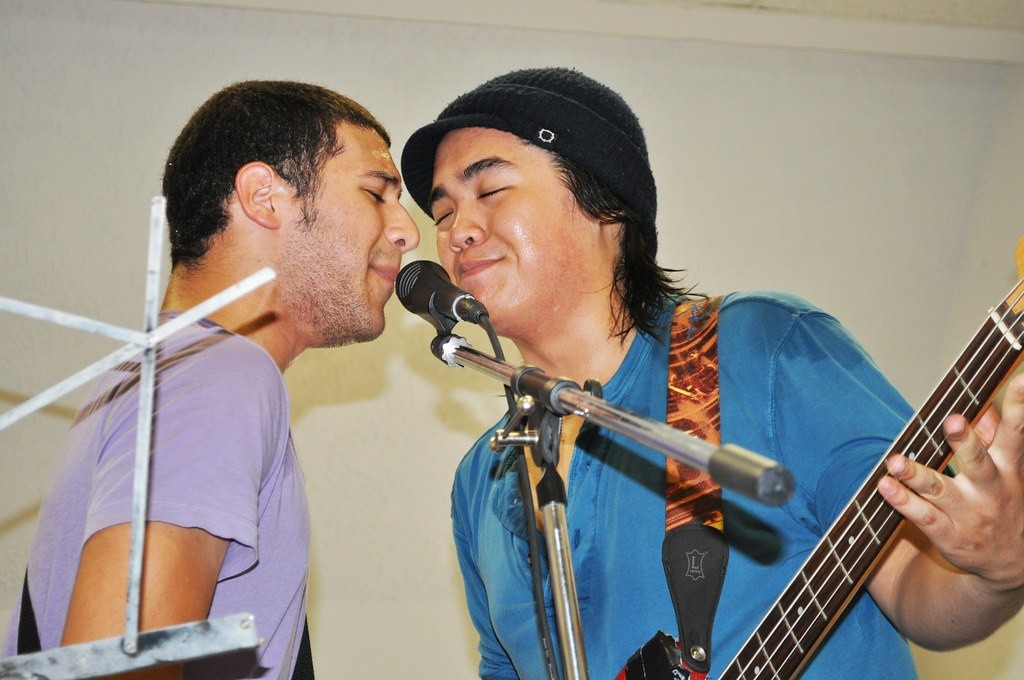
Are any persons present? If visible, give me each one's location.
[0,79,420,680]
[399,68,1024,680]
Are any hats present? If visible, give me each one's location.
[400,66,659,262]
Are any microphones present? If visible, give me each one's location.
[396,260,489,324]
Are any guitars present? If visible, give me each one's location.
[715,239,1024,680]
